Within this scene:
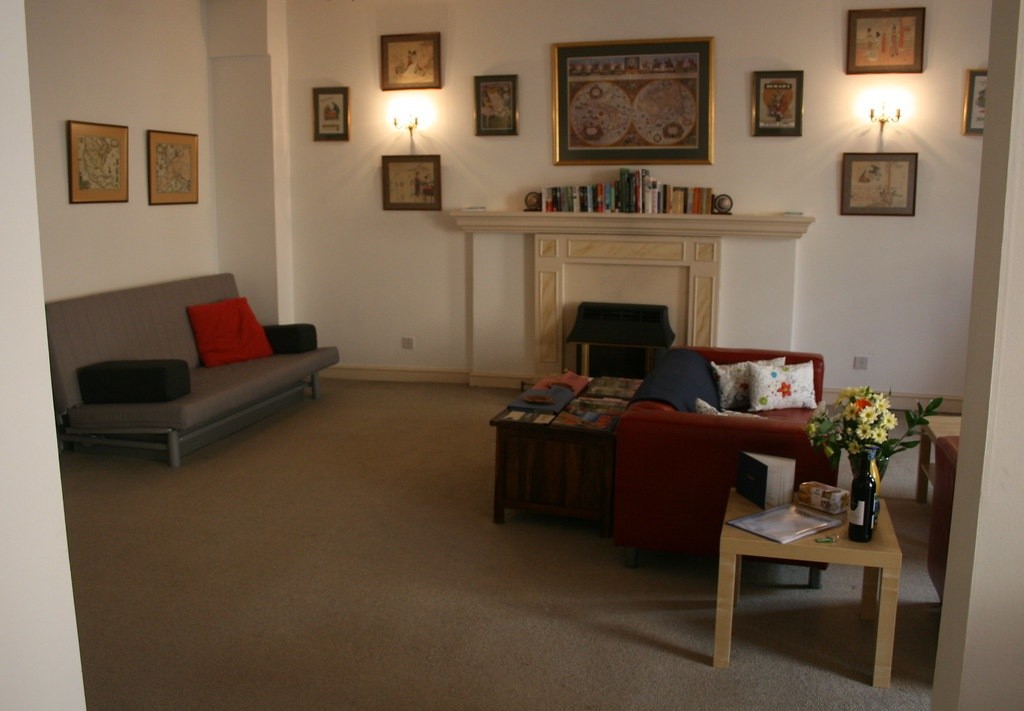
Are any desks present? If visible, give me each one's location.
[488,374,645,525]
[917,414,962,553]
[711,484,903,689]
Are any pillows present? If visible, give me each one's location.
[76,359,191,403]
[263,324,317,354]
[748,360,818,411]
[708,354,788,411]
[694,396,771,419]
[186,295,273,369]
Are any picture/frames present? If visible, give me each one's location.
[845,6,927,75]
[551,36,715,165]
[379,29,442,91]
[146,129,200,206]
[68,120,129,205]
[841,152,917,218]
[749,70,803,137]
[381,154,442,213]
[473,73,519,137]
[961,69,987,136]
[311,86,350,143]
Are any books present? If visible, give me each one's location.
[735,449,796,509]
[504,371,643,433]
[540,166,714,214]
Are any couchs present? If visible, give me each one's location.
[612,346,841,561]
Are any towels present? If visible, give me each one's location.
[505,371,589,415]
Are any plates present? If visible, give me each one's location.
[547,380,575,392]
[569,81,632,147]
[524,395,555,404]
[633,80,697,146]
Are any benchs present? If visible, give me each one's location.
[45,273,340,469]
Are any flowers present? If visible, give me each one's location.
[802,385,943,463]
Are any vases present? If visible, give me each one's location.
[845,453,891,542]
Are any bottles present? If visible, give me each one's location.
[849,449,876,542]
[864,443,882,531]
